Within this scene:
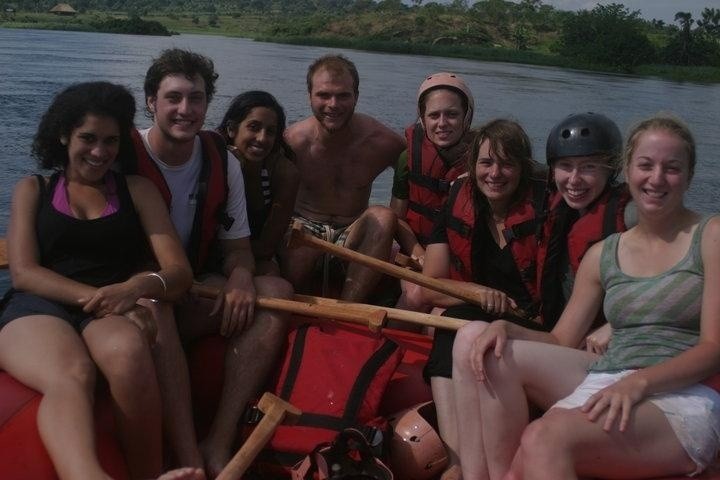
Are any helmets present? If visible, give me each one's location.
[417,72,475,134]
[382,400,449,479]
[545,112,622,163]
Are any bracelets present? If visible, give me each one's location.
[145,273,167,304]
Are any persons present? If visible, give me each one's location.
[385,69,487,318]
[213,86,302,283]
[415,115,548,479]
[132,46,298,480]
[0,78,210,480]
[275,52,410,301]
[449,110,640,479]
[465,115,719,477]
